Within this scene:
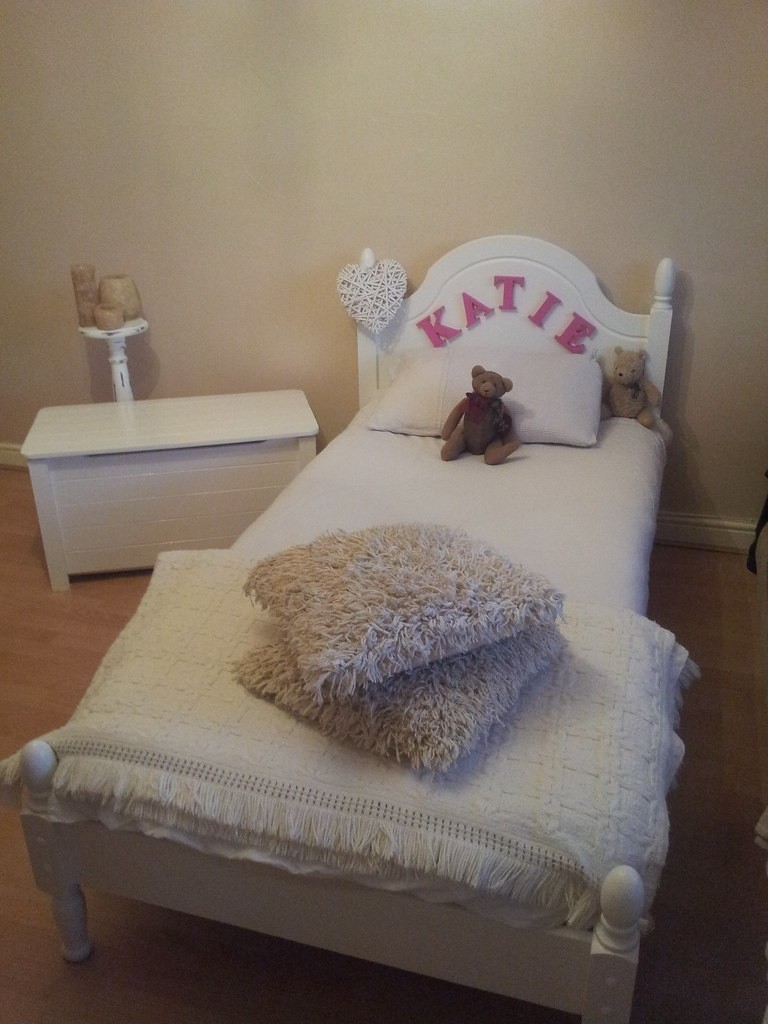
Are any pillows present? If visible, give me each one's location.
[361,347,603,447]
[232,522,570,773]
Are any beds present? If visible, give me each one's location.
[0,235,702,1024]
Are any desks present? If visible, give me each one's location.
[79,318,149,402]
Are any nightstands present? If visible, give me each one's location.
[20,390,320,592]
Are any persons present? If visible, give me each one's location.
[601,346,662,428]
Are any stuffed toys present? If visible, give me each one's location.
[441,365,522,466]
